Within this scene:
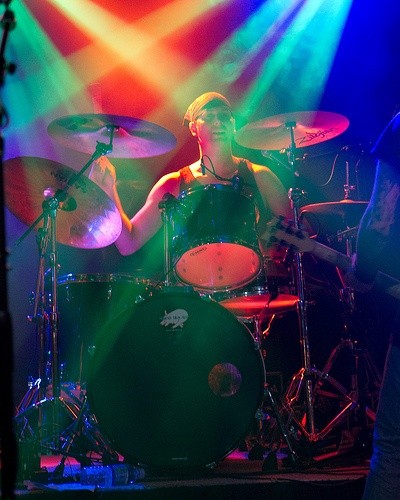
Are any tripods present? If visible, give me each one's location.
[10,138,117,475]
[246,120,377,467]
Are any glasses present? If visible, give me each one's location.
[200,112,231,122]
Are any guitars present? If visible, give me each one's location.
[253,206,400,300]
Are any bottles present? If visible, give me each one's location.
[82,463,146,487]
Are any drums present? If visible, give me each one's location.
[160,181,258,291]
[213,256,300,319]
[59,271,155,334]
[77,288,268,470]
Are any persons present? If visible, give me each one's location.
[88,90,313,461]
[342,112,400,500]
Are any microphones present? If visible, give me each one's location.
[260,149,303,178]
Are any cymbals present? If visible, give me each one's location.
[47,114,176,158]
[2,156,122,249]
[234,111,349,148]
[302,199,372,211]
